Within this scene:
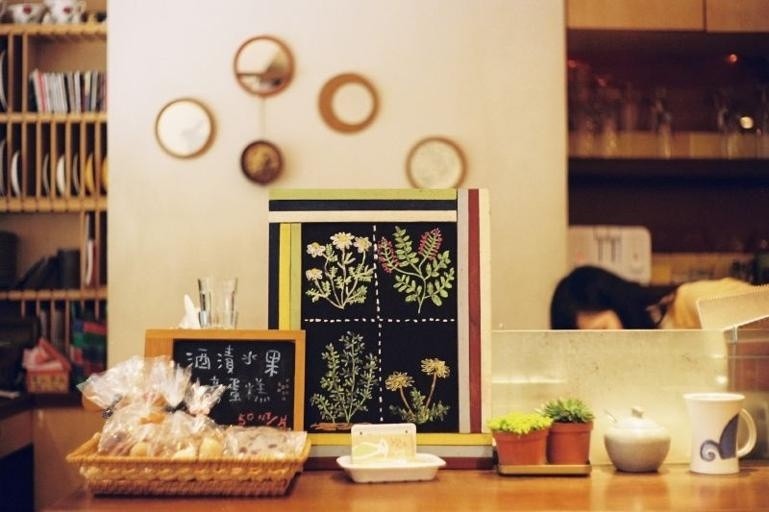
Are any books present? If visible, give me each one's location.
[27,67,106,111]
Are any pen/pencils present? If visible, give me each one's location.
[198,278,237,327]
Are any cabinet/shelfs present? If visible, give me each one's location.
[0,21,109,402]
[563,1,769,287]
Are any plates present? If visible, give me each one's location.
[0,141,108,199]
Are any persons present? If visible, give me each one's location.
[550,264,754,329]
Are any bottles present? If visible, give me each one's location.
[603,406,671,473]
[655,112,672,158]
[600,105,620,157]
[572,107,595,155]
[718,113,739,157]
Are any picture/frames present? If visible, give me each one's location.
[267,187,495,469]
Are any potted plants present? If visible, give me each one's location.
[486,405,554,467]
[538,396,595,467]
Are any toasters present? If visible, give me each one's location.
[567,226,651,285]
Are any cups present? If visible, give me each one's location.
[194,273,238,328]
[41,0,87,23]
[7,0,44,26]
[684,391,758,477]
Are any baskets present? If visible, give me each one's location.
[66,431,313,497]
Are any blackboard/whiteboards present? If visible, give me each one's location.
[172,330,306,431]
